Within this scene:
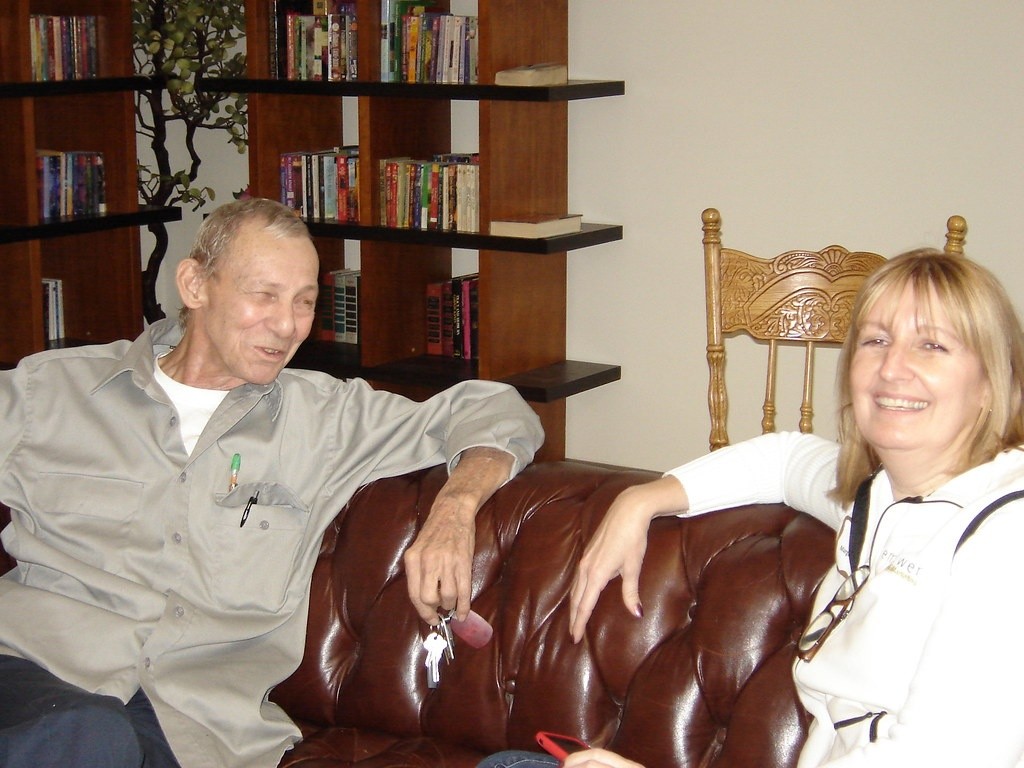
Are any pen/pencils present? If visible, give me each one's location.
[230,452,241,492]
[239,491,259,527]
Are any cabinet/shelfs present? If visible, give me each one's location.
[0,0,624,458]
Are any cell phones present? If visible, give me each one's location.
[535,731,589,764]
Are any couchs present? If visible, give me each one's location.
[268,457,837,768]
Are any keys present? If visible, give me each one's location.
[424,623,455,684]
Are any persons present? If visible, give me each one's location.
[475,247,1024,768]
[0,198,546,768]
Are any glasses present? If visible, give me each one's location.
[794,565,872,662]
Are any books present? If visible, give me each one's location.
[280,145,360,222]
[379,153,479,233]
[426,273,479,360]
[30,15,97,81]
[38,151,107,218]
[268,0,357,82]
[494,62,567,86]
[381,0,479,84]
[490,212,583,239]
[315,268,361,344]
[42,278,65,340]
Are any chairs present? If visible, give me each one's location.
[702,208,967,455]
